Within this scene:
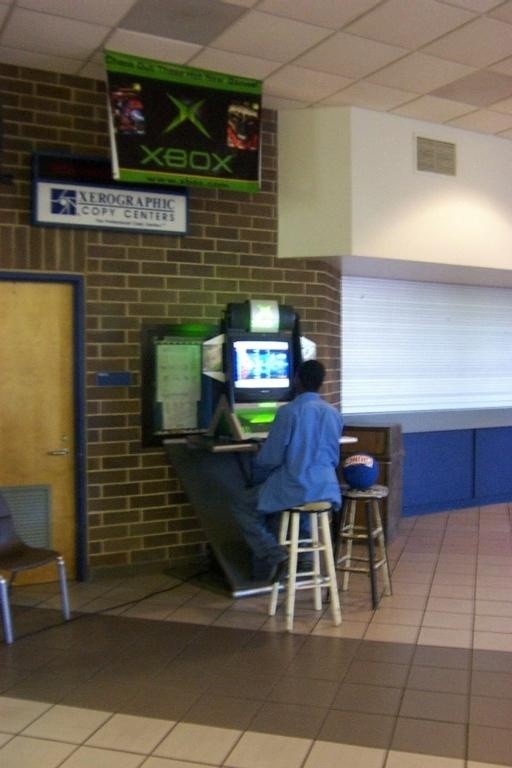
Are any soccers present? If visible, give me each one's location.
[342,453,380,488]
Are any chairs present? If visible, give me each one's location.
[0,499,72,646]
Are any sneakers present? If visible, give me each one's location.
[269,557,313,585]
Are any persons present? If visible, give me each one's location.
[230,359,343,584]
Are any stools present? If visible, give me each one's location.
[270,498,343,630]
[342,483,392,608]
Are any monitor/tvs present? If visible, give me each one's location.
[231,337,292,401]
